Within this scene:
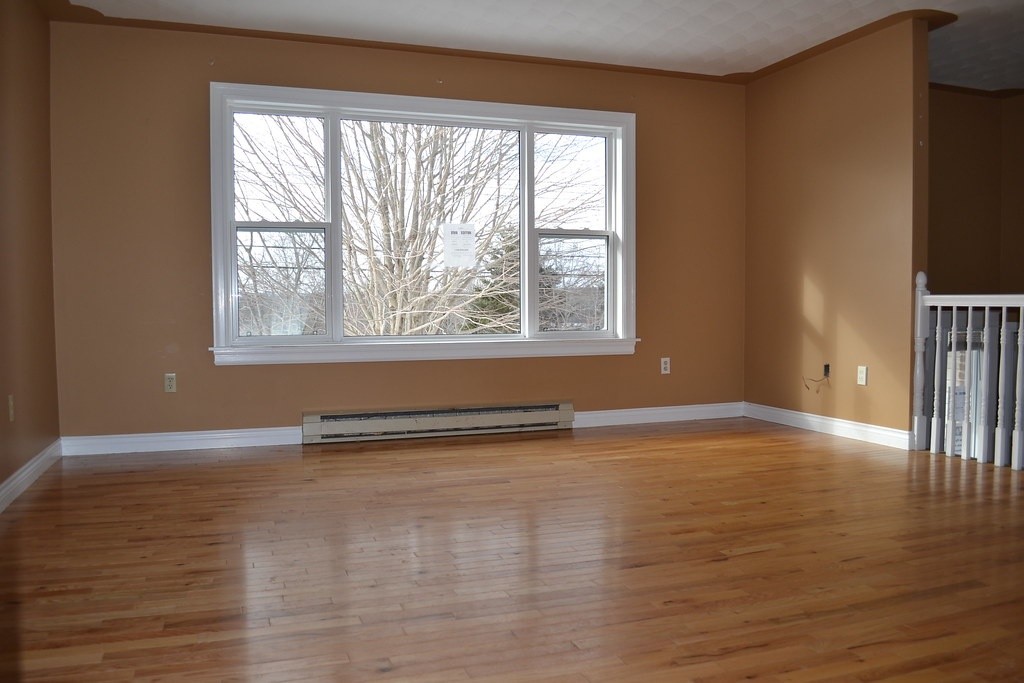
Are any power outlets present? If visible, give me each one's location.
[164,374,175,393]
[661,357,670,374]
[857,367,867,384]
[9,396,15,422]
